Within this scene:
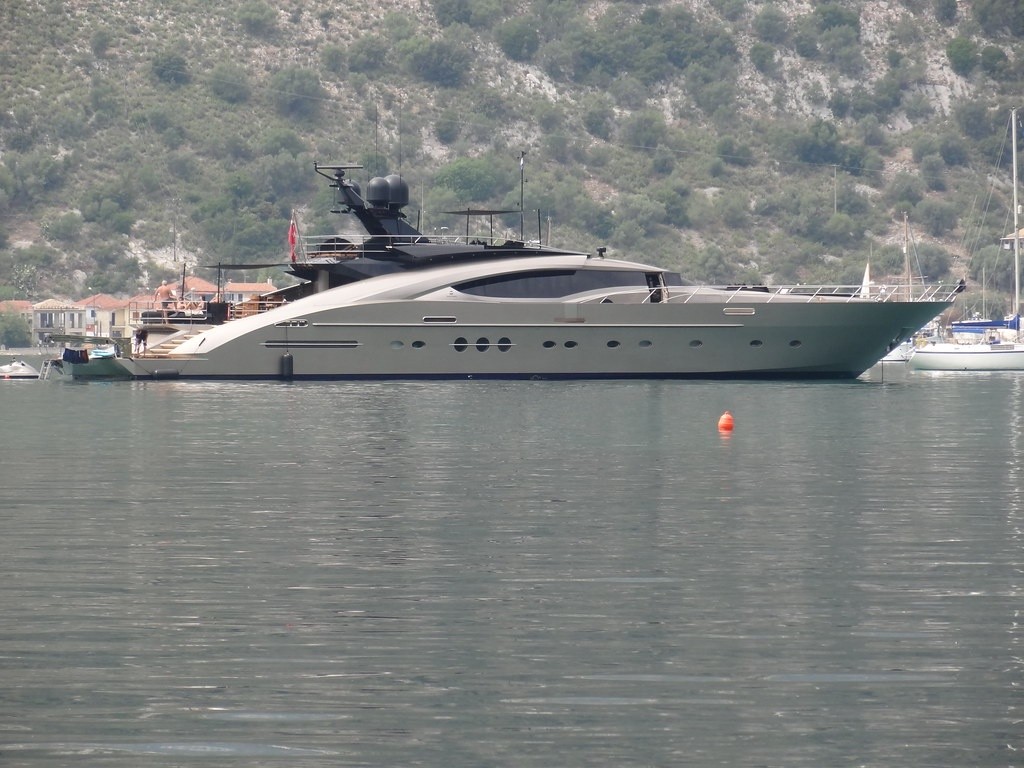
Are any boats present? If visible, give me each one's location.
[0,359,41,376]
[38,89,974,380]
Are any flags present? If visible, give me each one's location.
[288,209,299,263]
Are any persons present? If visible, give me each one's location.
[154,280,178,325]
[175,292,222,312]
[134,329,148,358]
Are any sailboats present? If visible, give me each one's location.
[857,104,1024,371]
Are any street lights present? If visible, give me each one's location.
[88,286,95,335]
